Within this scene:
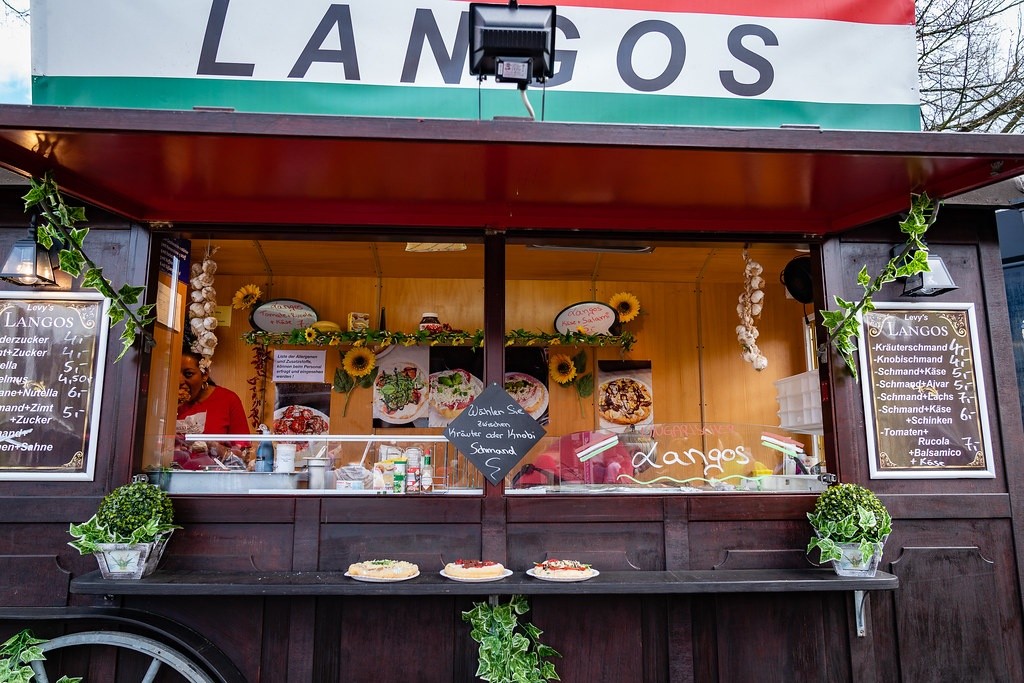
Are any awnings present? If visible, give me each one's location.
[0,104,1024,240]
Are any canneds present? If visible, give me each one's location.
[420,312,441,332]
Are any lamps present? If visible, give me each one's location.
[0,212,61,289]
[891,238,961,304]
[468,0,556,122]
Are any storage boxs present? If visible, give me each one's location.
[775,370,821,432]
[348,311,369,332]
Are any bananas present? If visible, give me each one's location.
[309,321,342,332]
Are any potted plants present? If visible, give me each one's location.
[808,485,892,574]
[62,475,180,582]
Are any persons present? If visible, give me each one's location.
[174,346,252,471]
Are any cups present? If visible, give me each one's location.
[302,456,330,489]
[393,459,406,494]
[276,443,296,473]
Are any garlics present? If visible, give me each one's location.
[735,261,768,371]
[188,260,218,369]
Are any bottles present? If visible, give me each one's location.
[420,313,441,332]
[421,449,433,493]
[255,429,274,472]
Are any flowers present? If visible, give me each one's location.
[230,280,641,417]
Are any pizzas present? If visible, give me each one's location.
[428,368,480,418]
[503,373,544,412]
[374,361,429,420]
[597,378,652,424]
[533,559,593,578]
[443,559,504,578]
[348,560,419,579]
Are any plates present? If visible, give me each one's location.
[525,568,600,582]
[598,377,653,434]
[344,571,421,582]
[429,370,484,428]
[275,406,329,424]
[439,569,514,583]
[374,357,429,425]
[505,371,549,421]
